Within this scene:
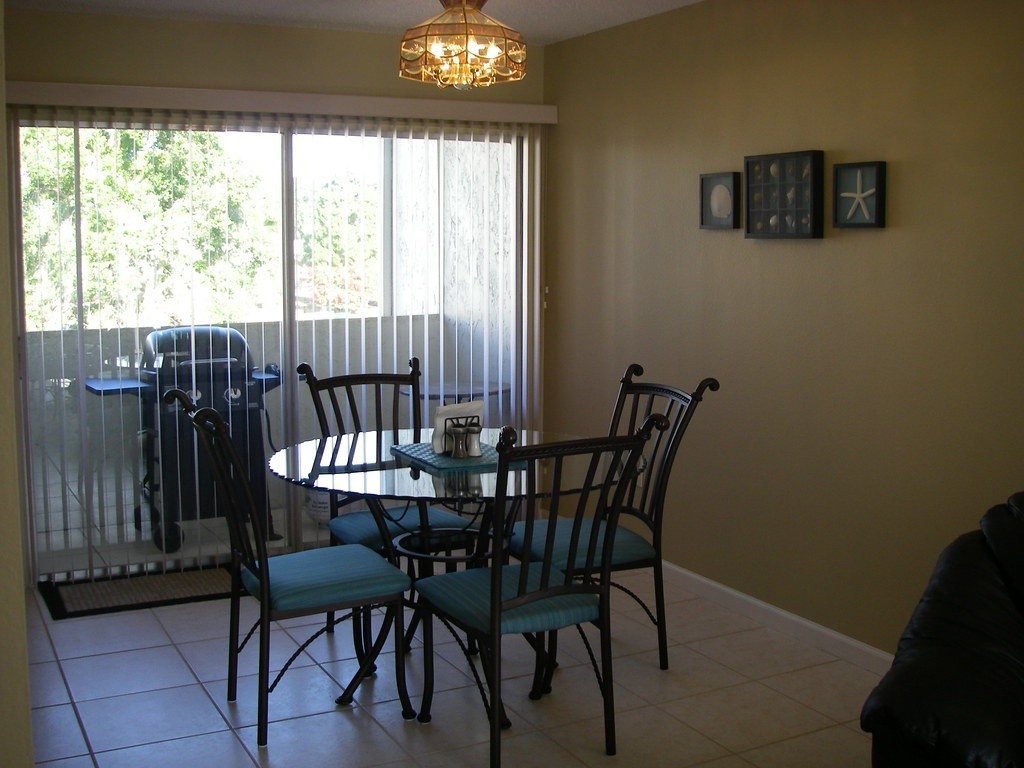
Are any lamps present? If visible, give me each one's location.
[399,0,528,91]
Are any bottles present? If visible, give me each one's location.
[467,423,483,457]
[451,423,467,458]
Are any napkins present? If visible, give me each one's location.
[432,400,484,453]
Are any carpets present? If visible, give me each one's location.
[38,563,252,620]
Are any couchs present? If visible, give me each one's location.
[861,492,1024,768]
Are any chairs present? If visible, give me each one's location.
[490,364,719,670]
[297,357,478,655]
[164,388,416,747]
[414,413,671,767]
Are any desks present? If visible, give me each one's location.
[398,378,511,406]
[269,428,647,728]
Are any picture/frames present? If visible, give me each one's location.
[699,171,740,231]
[833,161,887,229]
[743,150,823,240]
